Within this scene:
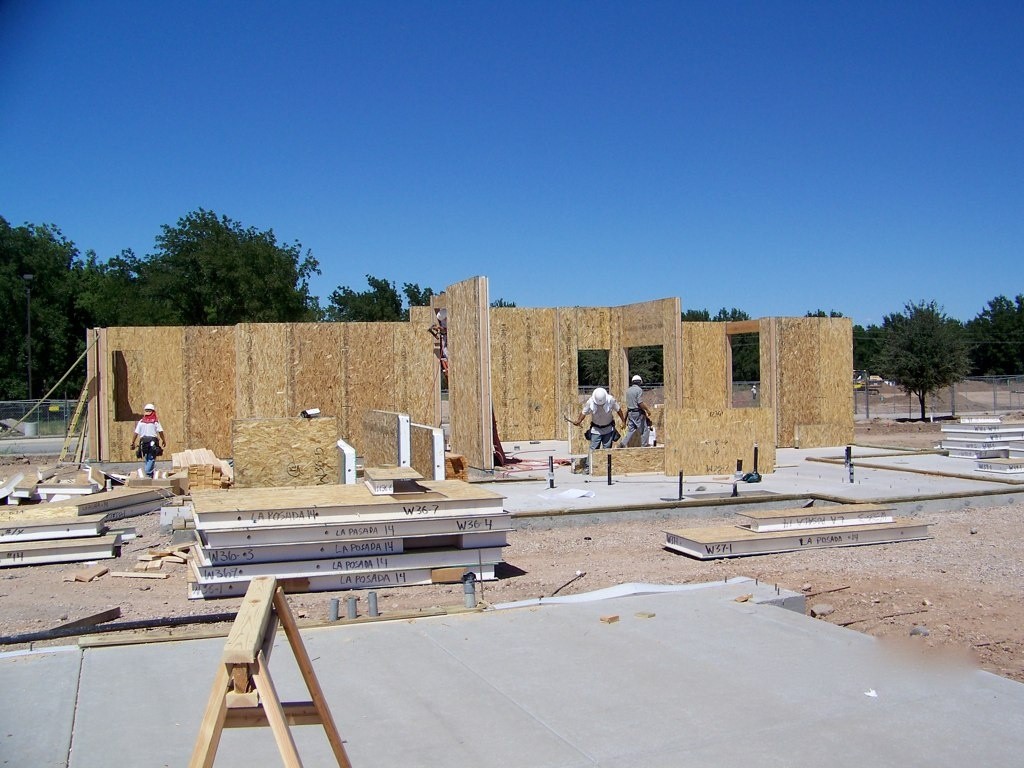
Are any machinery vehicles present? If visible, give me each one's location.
[853,370,883,396]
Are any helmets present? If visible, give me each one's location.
[632,375,642,383]
[592,388,608,406]
[144,404,154,411]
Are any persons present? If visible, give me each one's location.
[617,375,652,448]
[750,385,758,399]
[129,404,166,479]
[573,388,626,475]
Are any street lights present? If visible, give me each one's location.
[22,273,39,402]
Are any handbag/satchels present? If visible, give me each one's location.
[584,429,591,440]
[613,430,621,442]
[156,446,164,457]
[135,446,142,460]
[646,416,653,426]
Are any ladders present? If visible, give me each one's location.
[58,384,88,462]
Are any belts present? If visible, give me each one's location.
[628,409,639,412]
